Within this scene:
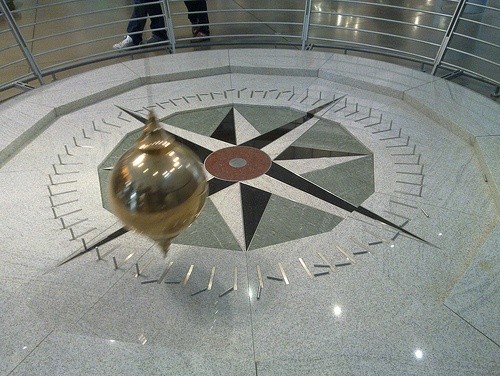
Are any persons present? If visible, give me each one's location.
[184,0,211,43]
[112,0,168,50]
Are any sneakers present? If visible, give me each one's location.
[113,35,141,50]
[190,24,210,42]
[143,35,168,44]
